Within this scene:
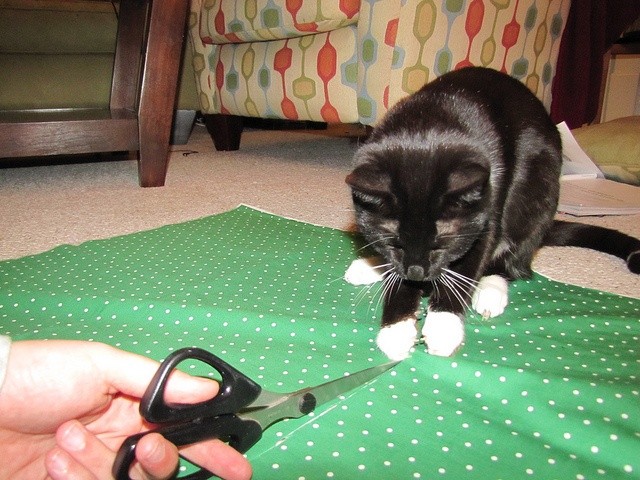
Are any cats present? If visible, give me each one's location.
[342,65,640,364]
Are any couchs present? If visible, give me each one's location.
[0,1,198,143]
[190,0,570,152]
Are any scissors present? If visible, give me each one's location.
[111,347,402,480]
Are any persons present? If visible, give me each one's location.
[0,333,250,480]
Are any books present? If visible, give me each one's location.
[554,120,638,216]
